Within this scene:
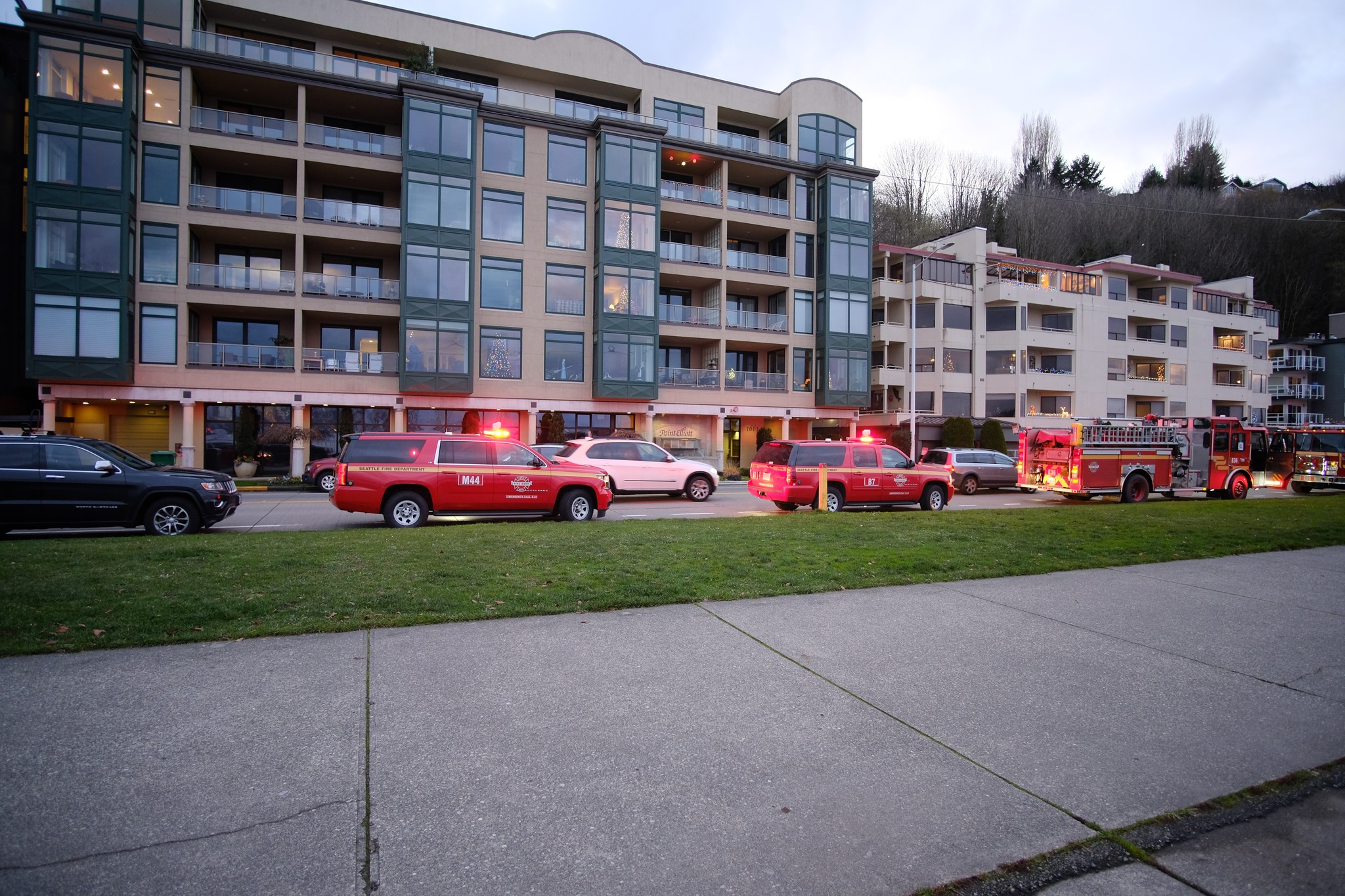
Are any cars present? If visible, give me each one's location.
[300,457,338,493]
[205,428,238,454]
[498,442,565,467]
[252,433,334,465]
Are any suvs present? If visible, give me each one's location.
[551,437,721,502]
[0,428,242,538]
[329,432,618,526]
[917,447,1040,495]
[746,429,955,512]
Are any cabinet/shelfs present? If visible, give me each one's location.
[704,225,721,266]
[1073,279,1091,291]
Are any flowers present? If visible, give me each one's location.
[232,405,261,466]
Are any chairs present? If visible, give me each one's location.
[338,290,373,299]
[1286,387,1294,396]
[743,380,753,389]
[219,352,238,366]
[278,279,295,293]
[325,359,339,372]
[760,321,784,332]
[683,317,696,324]
[251,354,272,369]
[726,316,739,328]
[331,215,378,227]
[344,352,383,373]
[860,452,873,466]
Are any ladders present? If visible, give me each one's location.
[1069,423,1182,447]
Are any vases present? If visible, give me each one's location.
[233,462,258,479]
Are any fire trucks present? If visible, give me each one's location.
[1282,421,1345,493]
[1015,414,1297,504]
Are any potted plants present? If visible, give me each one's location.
[282,349,294,370]
[693,255,707,264]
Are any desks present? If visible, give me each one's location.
[301,358,325,371]
[195,197,210,208]
[238,362,250,368]
[345,292,364,298]
[696,318,708,325]
[700,377,717,386]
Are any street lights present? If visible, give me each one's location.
[910,243,955,464]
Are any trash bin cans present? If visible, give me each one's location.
[149,450,177,467]
